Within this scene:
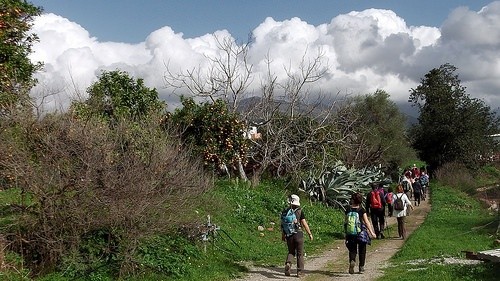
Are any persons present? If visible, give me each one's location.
[391,185,414,239]
[399,164,429,215]
[281,194,313,277]
[378,184,395,218]
[343,192,377,274]
[365,182,386,240]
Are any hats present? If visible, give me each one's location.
[287,195,301,206]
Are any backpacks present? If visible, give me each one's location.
[401,181,410,193]
[345,208,363,236]
[283,207,301,235]
[370,190,381,208]
[393,193,405,211]
[386,192,393,204]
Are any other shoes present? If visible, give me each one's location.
[418,205,419,206]
[399,235,401,237]
[298,271,304,277]
[349,261,355,274]
[402,237,405,240]
[380,231,386,238]
[285,263,291,276]
[388,213,391,217]
[359,265,365,273]
[415,202,416,206]
[376,236,380,239]
